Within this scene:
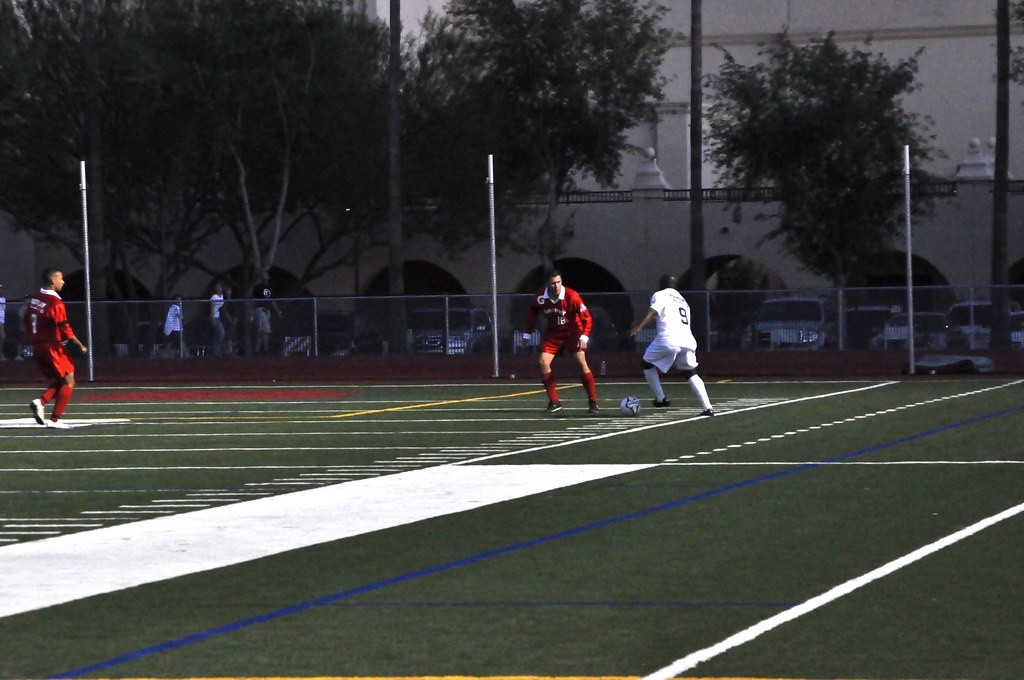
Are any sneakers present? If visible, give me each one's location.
[697,409,714,417]
[653,397,671,407]
[30,398,45,425]
[545,400,562,413]
[586,400,599,413]
[47,418,70,429]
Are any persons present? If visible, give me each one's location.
[210,285,283,360]
[26,266,89,431]
[628,273,715,417]
[160,295,184,358]
[538,271,600,414]
[0,285,36,363]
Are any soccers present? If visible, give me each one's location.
[620,396,641,416]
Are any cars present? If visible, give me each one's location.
[871,311,948,350]
[588,305,619,355]
[316,311,389,356]
[941,300,1020,349]
[410,307,492,356]
[1010,310,1024,350]
[744,297,839,351]
